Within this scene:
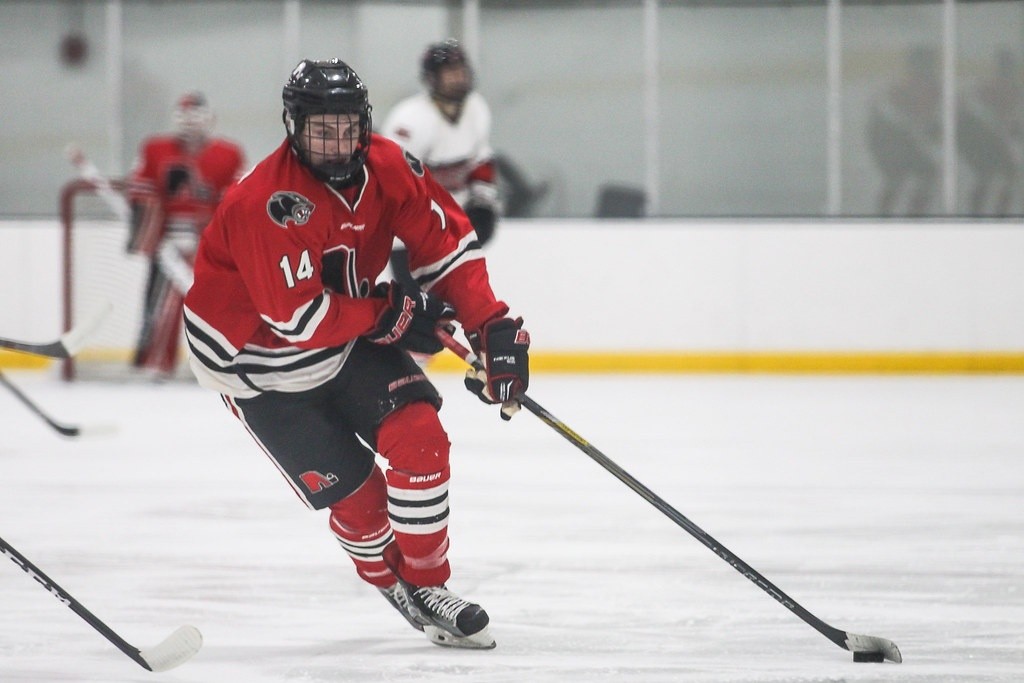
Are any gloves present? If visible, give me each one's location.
[464,322,529,420]
[472,206,496,246]
[361,280,458,355]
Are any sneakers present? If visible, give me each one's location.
[398,573,495,650]
[381,580,441,647]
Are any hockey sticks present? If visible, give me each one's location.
[436,325,904,666]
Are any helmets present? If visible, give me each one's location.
[281,57,374,188]
[423,39,474,103]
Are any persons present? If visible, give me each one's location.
[184,58,528,648]
[378,37,500,371]
[129,93,250,383]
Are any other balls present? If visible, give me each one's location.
[852,650,887,665]
[1,537,203,675]
[0,371,82,437]
[65,142,195,296]
